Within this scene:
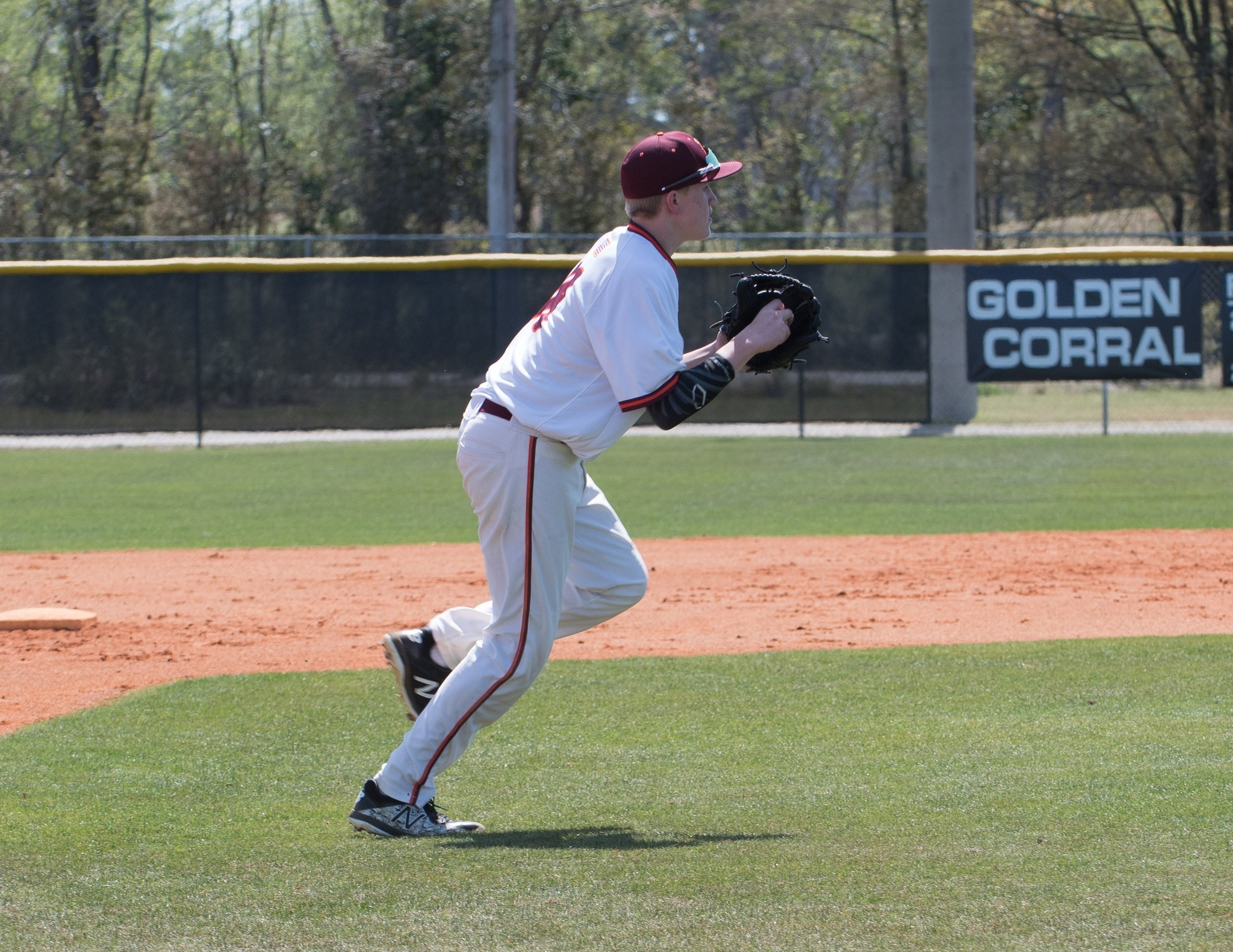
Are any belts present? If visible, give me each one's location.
[479,398,567,445]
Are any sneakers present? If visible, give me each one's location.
[348,777,485,838]
[377,626,452,721]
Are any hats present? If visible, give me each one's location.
[620,131,743,199]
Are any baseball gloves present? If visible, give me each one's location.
[707,257,830,377]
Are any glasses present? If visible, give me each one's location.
[660,148,720,191]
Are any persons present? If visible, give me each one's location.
[348,133,822,841]
